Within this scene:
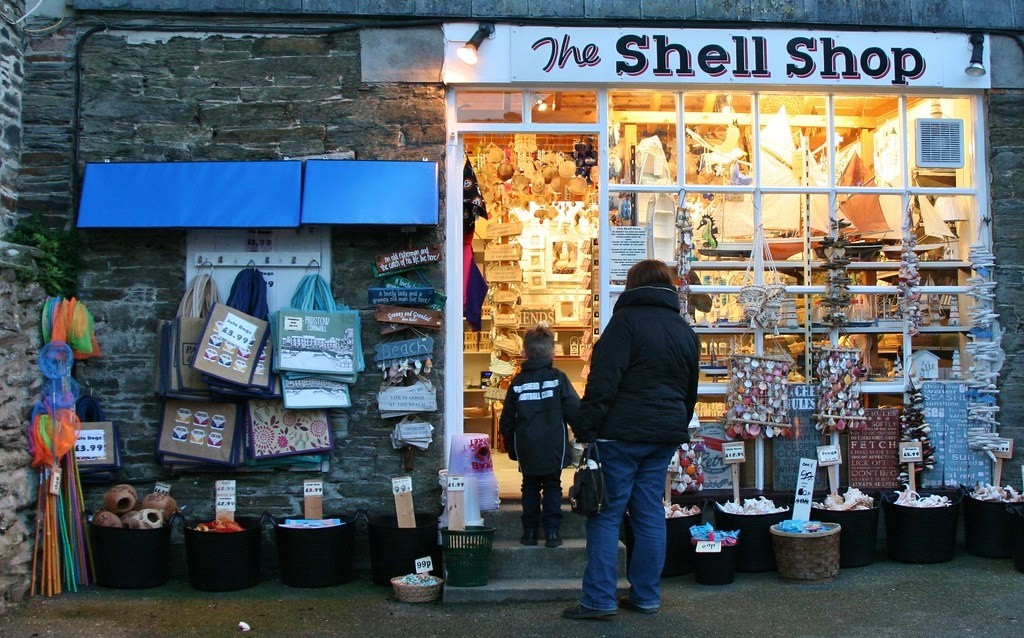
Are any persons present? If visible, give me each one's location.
[563,259,699,618]
[499,327,582,547]
[686,269,712,349]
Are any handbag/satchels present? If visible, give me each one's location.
[153,269,366,475]
[568,440,607,516]
[68,395,125,485]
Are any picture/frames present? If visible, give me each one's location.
[528,230,581,291]
[555,296,580,322]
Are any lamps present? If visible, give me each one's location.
[456,25,491,65]
[965,32,985,78]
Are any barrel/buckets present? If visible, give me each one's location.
[441,432,500,511]
[83,509,177,589]
[436,475,484,546]
[175,510,269,592]
[964,484,1024,573]
[361,508,445,586]
[793,492,882,569]
[266,508,362,588]
[622,498,708,579]
[881,489,965,564]
[708,496,793,574]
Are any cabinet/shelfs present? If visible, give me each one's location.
[464,316,591,451]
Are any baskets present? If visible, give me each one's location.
[391,575,444,602]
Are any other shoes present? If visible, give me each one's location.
[564,603,617,618]
[521,529,563,547]
[618,595,660,614]
